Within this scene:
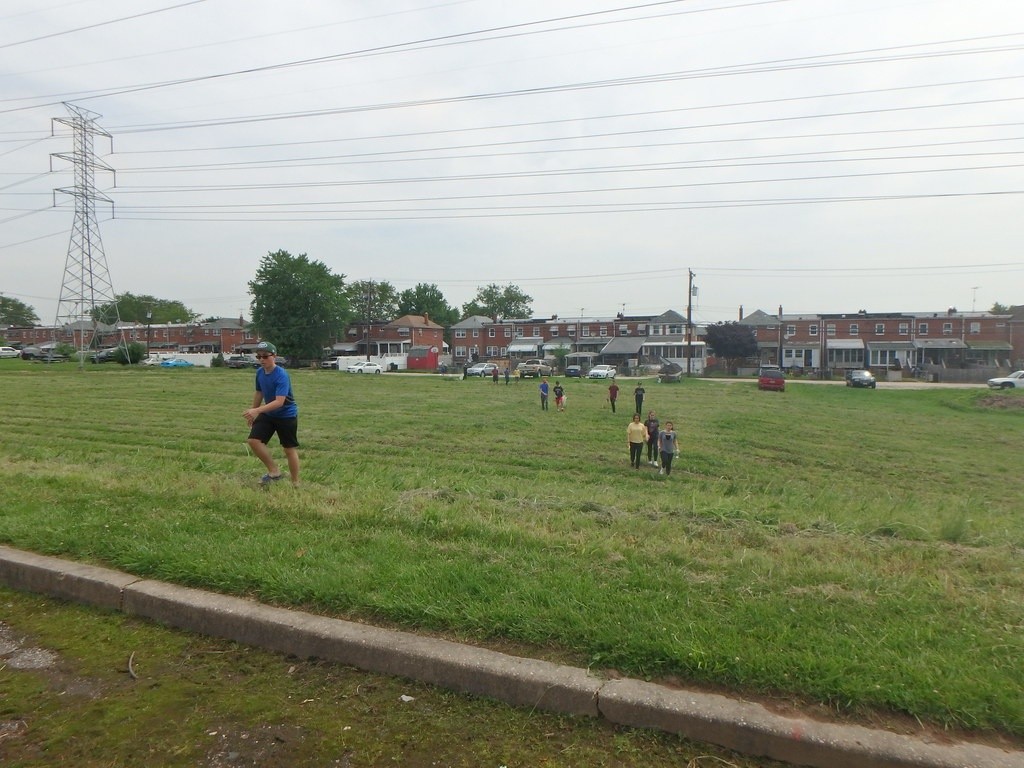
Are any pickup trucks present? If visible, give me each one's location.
[517,358,553,379]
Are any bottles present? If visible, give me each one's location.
[676,448,679,458]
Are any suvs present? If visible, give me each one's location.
[227,355,287,370]
[321,356,346,368]
[467,363,498,378]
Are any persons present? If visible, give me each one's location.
[463,364,467,380]
[491,367,499,384]
[242,342,299,485]
[627,413,646,470]
[608,381,619,413]
[538,379,550,411]
[633,382,645,415]
[504,368,510,385]
[440,361,445,375]
[657,421,679,476]
[514,368,520,383]
[644,412,660,467]
[553,381,566,410]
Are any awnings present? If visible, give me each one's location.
[826,339,864,349]
[323,342,358,351]
[98,340,178,348]
[33,340,59,349]
[965,341,1013,351]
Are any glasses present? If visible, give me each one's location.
[256,354,274,359]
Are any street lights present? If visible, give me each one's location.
[146,309,152,358]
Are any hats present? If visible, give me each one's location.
[256,341,277,355]
[638,381,642,384]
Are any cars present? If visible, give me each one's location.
[758,370,785,392]
[160,358,194,369]
[87,343,121,365]
[0,341,77,364]
[657,363,683,383]
[347,362,384,375]
[988,370,1024,391]
[588,364,617,380]
[845,369,876,389]
[564,365,583,379]
[140,357,167,369]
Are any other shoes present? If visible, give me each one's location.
[654,460,658,466]
[261,472,283,484]
[659,468,664,474]
[648,461,652,464]
